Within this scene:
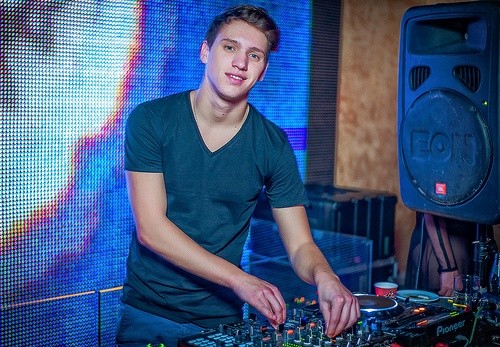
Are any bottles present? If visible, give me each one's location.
[466,241,495,344]
[490,254,499,295]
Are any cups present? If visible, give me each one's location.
[374,282,398,299]
[452,274,481,310]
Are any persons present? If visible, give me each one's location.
[115,5,362,347]
[404,211,500,305]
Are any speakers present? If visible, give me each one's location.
[398,2,499,225]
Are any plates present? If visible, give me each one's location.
[396,289,440,304]
